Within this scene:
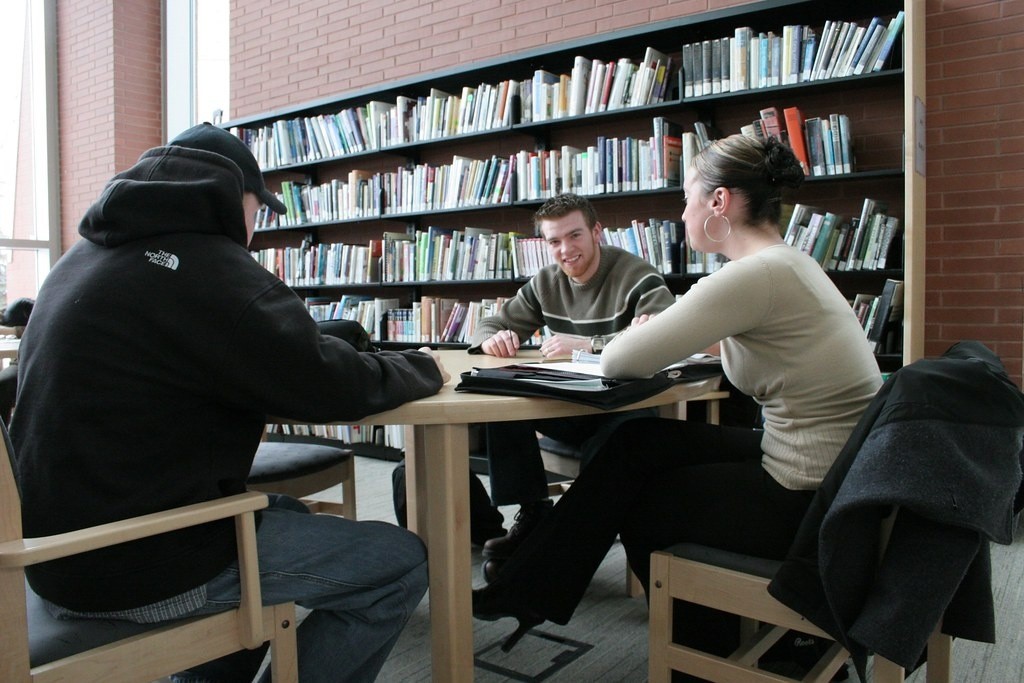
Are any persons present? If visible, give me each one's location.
[468,132,884,681]
[9,122,453,682]
[467,191,678,585]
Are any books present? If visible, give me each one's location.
[223,11,907,362]
[265,424,408,451]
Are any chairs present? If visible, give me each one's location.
[0,415,302,683]
[647,344,978,683]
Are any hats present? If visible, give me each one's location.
[168,121,286,216]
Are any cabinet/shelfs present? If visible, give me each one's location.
[210,0,926,486]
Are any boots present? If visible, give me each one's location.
[483,499,554,558]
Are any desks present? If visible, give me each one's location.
[266,348,721,683]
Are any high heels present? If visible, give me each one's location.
[471,576,546,650]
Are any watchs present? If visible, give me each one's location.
[591,334,606,355]
